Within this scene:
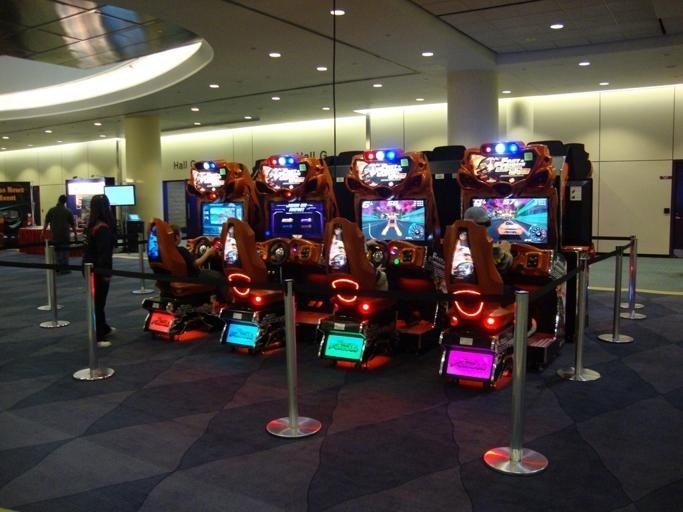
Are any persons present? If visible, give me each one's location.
[81,194,118,348]
[169,223,226,305]
[40,194,78,276]
[450,207,514,288]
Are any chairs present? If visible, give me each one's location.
[323,216,398,316]
[219,217,284,309]
[443,219,515,331]
[145,215,220,308]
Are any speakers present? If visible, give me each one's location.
[122,221,145,253]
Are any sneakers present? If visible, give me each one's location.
[96,327,116,347]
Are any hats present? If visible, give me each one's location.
[465,206,489,224]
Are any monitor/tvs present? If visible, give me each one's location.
[104,185,136,206]
[65,177,106,217]
[268,199,324,240]
[469,196,549,248]
[201,202,243,236]
[358,199,427,245]
